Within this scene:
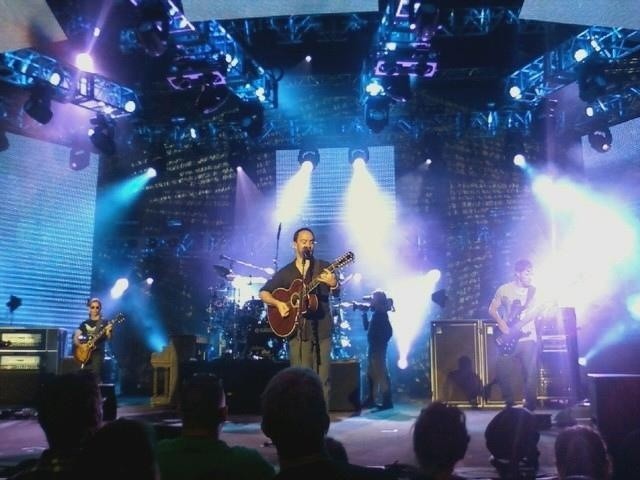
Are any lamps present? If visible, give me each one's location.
[124,100,563,184]
[464,26,640,166]
[359,0,442,137]
[120,1,279,139]
[0,46,145,172]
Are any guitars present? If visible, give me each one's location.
[494,312,538,355]
[75,314,125,362]
[267,252,354,337]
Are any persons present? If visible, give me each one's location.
[553,426,612,480]
[73,298,112,379]
[488,260,539,411]
[9,368,276,479]
[259,229,341,414]
[412,401,470,480]
[485,407,541,479]
[362,291,393,409]
[261,367,422,480]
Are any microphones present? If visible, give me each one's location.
[302,245,312,260]
[276,223,282,240]
[84,298,91,306]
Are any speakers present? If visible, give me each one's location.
[586,373,640,445]
[429,321,483,410]
[483,320,531,408]
[98,384,117,421]
